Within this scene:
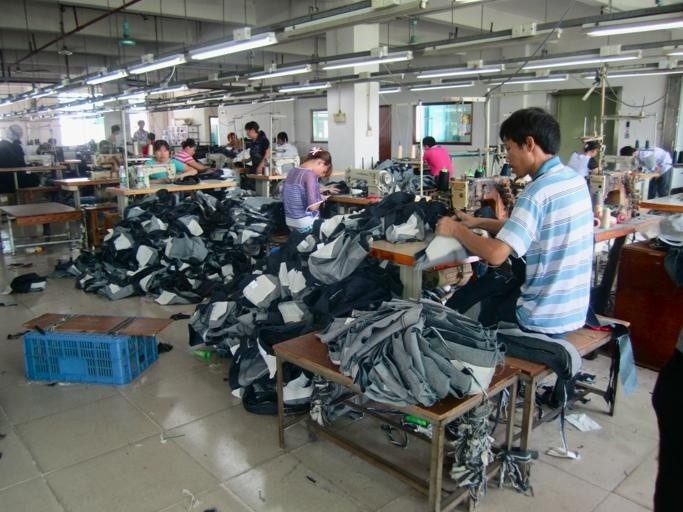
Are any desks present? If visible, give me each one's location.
[1,157,683,373]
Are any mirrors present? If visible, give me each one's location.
[208,115,218,146]
[411,100,475,146]
[309,107,328,144]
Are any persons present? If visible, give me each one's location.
[422,136,453,182]
[0,120,300,185]
[282,146,341,239]
[620,146,673,199]
[435,107,594,339]
[567,141,601,193]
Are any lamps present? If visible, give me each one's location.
[0,0,683,123]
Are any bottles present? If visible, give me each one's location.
[136,164,144,189]
[119,166,128,191]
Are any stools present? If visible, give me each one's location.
[270,330,523,512]
[495,314,631,452]
